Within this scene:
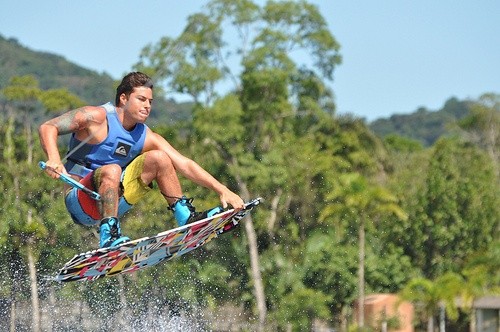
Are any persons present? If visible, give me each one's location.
[38,72,245,248]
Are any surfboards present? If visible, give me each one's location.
[58,198,265,283]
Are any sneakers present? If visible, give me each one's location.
[169,195,224,227]
[99,216,131,249]
